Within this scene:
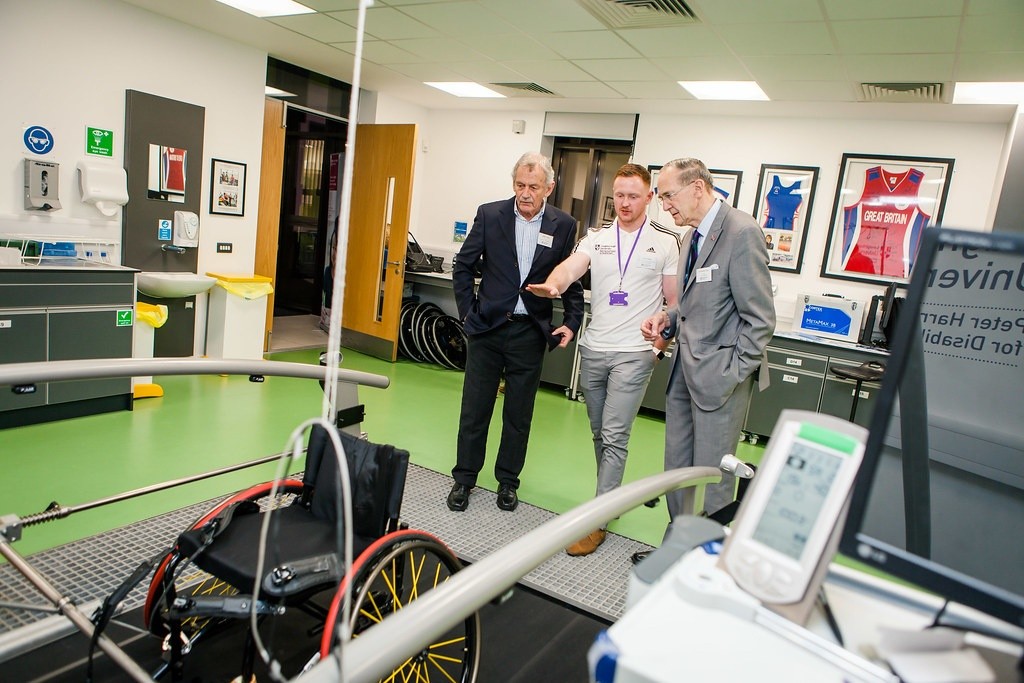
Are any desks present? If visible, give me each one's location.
[403,269,482,293]
[206,272,273,377]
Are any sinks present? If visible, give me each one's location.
[136,272,217,298]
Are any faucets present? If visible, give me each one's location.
[161,244,186,254]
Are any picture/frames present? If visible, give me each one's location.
[818,153,955,291]
[209,158,248,217]
[708,168,744,209]
[645,165,692,243]
[752,163,821,274]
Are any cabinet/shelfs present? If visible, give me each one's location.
[539,307,583,388]
[818,357,883,431]
[743,346,829,438]
[0,271,134,431]
[640,339,676,412]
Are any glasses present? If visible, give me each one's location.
[658,181,695,205]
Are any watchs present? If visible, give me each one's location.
[653,347,665,360]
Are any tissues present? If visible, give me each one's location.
[77,161,130,217]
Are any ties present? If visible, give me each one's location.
[683,230,700,291]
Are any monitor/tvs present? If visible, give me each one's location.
[838,225,1024,628]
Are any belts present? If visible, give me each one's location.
[508,314,530,321]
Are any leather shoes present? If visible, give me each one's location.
[497,483,518,510]
[447,483,471,511]
[631,551,653,564]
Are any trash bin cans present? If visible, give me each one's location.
[132,302,163,398]
[205,271,272,377]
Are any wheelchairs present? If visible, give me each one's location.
[86,401,482,683]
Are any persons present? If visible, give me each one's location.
[525,163,683,554]
[447,153,585,510]
[325,232,337,308]
[632,158,776,563]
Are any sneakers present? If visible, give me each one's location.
[566,529,605,556]
